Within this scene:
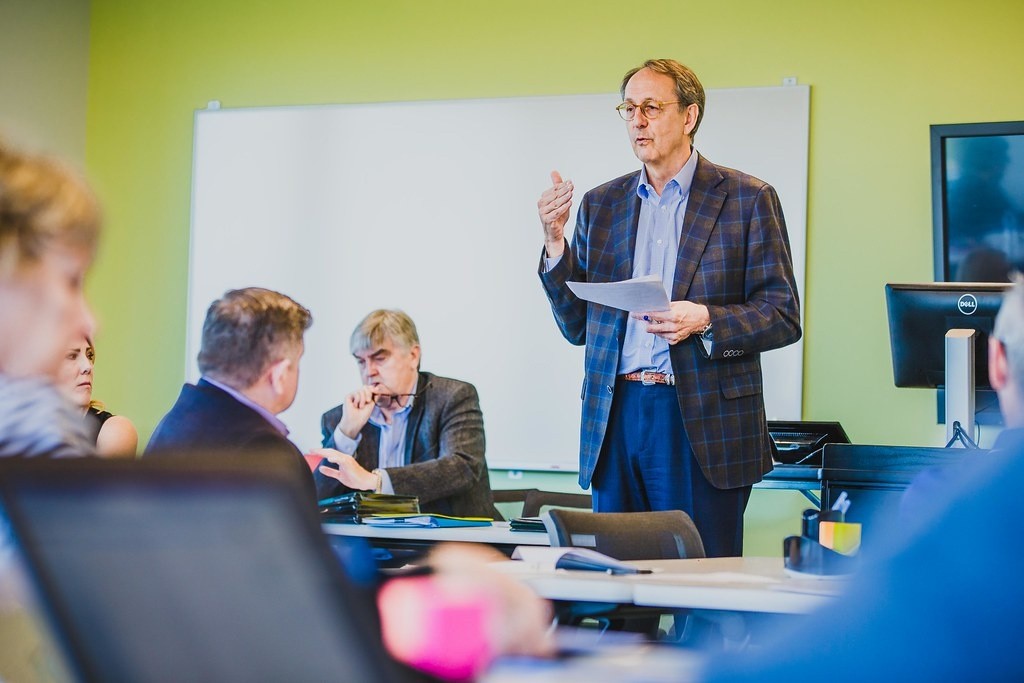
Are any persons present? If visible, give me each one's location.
[0,147,1024,683]
[536,60,803,652]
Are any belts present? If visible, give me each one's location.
[616,369,676,387]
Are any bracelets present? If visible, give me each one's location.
[371,470,383,492]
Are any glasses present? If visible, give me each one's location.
[616,99,687,121]
[372,382,433,408]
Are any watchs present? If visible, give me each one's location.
[700,322,712,341]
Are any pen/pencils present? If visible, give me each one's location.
[643,314,659,324]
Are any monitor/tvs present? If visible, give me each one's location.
[766,421,853,467]
[931,120,1024,427]
[886,283,1013,447]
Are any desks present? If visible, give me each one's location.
[498,552,855,613]
[327,518,548,547]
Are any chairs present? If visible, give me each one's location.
[549,507,708,639]
[520,491,593,519]
